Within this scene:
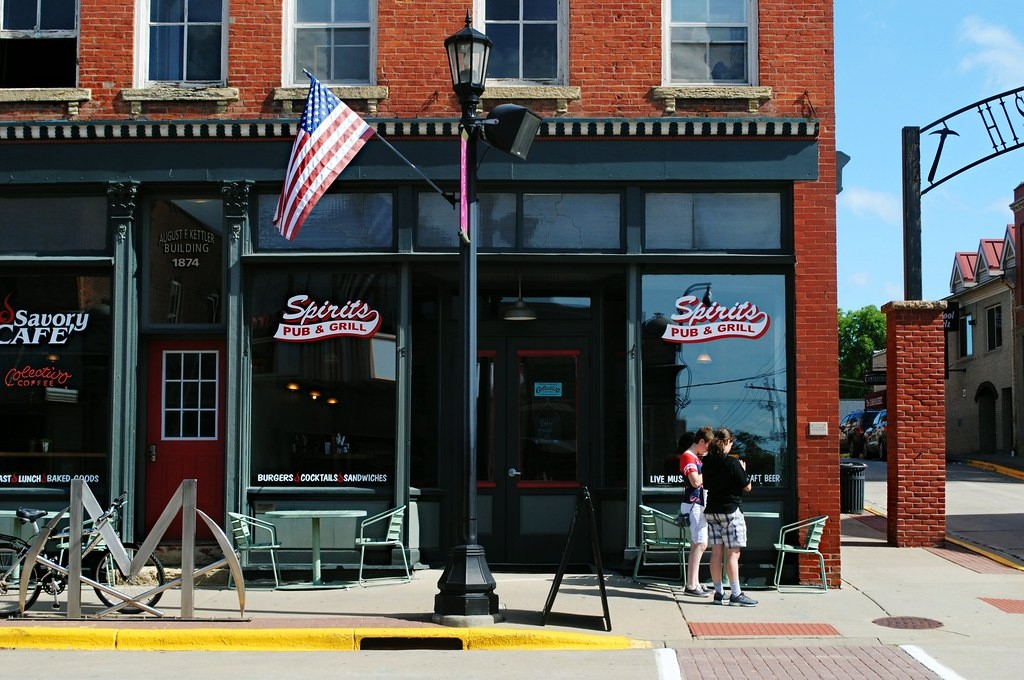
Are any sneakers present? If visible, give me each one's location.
[684,585,709,597]
[701,583,715,594]
[729,592,758,607]
[714,592,729,604]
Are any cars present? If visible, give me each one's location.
[839,409,887,462]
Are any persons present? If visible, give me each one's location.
[701,429,757,606]
[679,427,716,597]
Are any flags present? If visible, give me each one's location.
[271,76,376,242]
[342,197,391,304]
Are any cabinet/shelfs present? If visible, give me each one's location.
[278,427,392,486]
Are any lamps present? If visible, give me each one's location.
[968,320,976,325]
[327,396,339,408]
[310,391,320,399]
[287,383,300,392]
[503,273,536,320]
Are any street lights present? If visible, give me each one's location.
[434,9,543,617]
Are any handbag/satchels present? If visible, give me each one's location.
[677,513,689,527]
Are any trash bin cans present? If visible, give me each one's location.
[840,461,868,515]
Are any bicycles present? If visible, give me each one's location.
[0,489,166,619]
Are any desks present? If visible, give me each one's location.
[0,510,70,590]
[265,510,368,591]
[701,512,780,589]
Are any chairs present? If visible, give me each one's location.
[227,512,282,592]
[355,505,411,587]
[632,505,687,589]
[57,510,117,590]
[773,515,828,594]
